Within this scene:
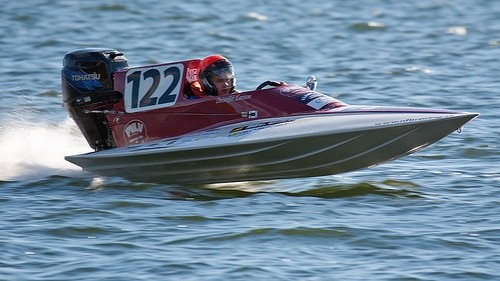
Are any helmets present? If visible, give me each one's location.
[196,55,235,96]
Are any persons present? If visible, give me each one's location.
[191,54,235,99]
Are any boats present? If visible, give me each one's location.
[60,48,481,183]
